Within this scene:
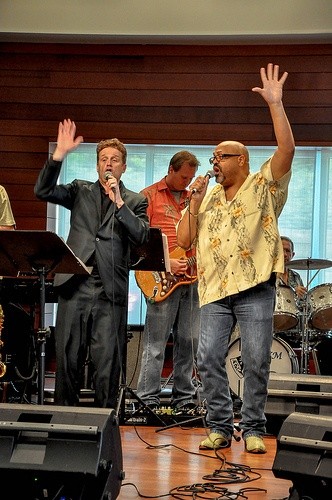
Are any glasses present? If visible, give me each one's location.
[209,154,240,166]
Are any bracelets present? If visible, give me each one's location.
[187,207,200,217]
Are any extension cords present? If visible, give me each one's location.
[147,413,205,427]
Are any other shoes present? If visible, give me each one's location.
[244,435,267,454]
[199,432,232,450]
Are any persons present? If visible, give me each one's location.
[34,118,149,410]
[137,151,202,410]
[176,63,295,454]
[0,185,16,231]
[275,236,307,298]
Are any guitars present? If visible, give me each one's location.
[135,246,197,302]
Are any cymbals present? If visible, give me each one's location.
[284,259,332,270]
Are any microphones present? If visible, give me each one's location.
[106,167,115,193]
[191,170,215,193]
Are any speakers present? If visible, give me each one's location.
[0,403,124,500]
[271,412,332,500]
[265,390,332,435]
[267,373,332,393]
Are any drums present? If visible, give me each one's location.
[273,285,299,331]
[225,336,299,401]
[307,283,332,329]
